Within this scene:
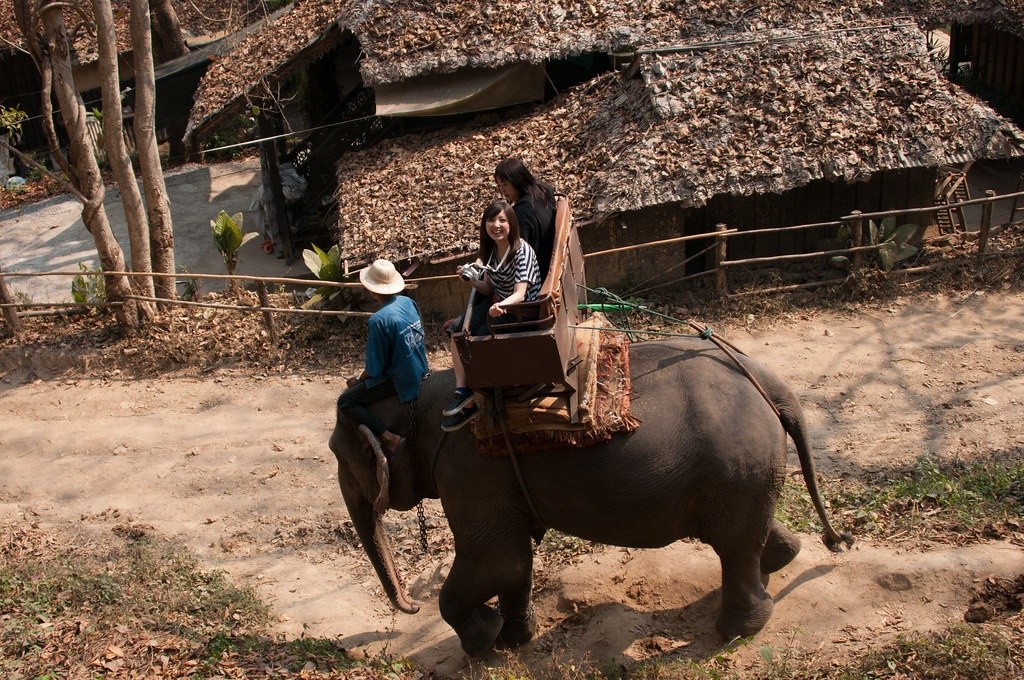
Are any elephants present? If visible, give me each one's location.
[326,333,841,664]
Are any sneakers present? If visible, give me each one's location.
[442,387,476,416]
[440,403,478,432]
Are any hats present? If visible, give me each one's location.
[359,258,405,295]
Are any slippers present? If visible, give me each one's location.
[387,437,406,475]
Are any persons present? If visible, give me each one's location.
[337,259,428,454]
[440,159,556,432]
